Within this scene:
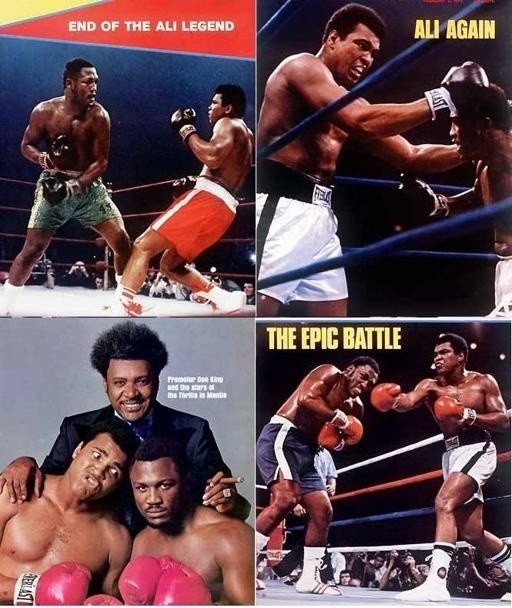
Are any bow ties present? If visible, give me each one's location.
[111,413,154,441]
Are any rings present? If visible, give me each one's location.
[222,487,231,499]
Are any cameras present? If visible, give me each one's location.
[76,266,83,270]
[355,552,364,559]
[398,556,407,564]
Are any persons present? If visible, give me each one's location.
[370,333,511,604]
[204,475,245,484]
[0,322,251,541]
[256,545,511,598]
[118,436,255,606]
[254,357,378,597]
[1,422,139,605]
[270,445,341,590]
[255,3,491,318]
[0,59,156,318]
[99,81,255,319]
[0,246,255,307]
[395,83,511,319]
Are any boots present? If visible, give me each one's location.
[112,274,151,316]
[297,546,343,597]
[397,548,451,602]
[499,559,511,601]
[0,279,25,315]
[193,280,245,315]
[256,530,268,591]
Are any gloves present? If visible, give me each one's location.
[12,563,123,606]
[118,554,213,606]
[38,134,77,170]
[172,175,195,197]
[400,173,449,222]
[40,176,83,204]
[371,381,402,411]
[172,108,199,143]
[437,396,476,429]
[316,408,364,450]
[425,60,487,122]
